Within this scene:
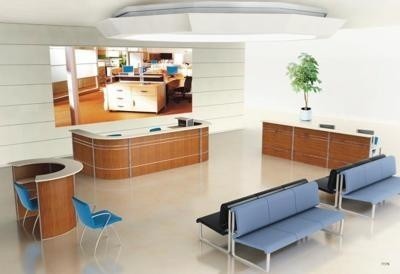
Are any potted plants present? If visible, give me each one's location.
[288,53,322,121]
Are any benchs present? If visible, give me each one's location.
[339,156,400,218]
[230,181,345,273]
[313,154,386,208]
[196,179,308,274]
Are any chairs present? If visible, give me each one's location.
[171,76,192,104]
[14,182,39,234]
[72,196,122,257]
